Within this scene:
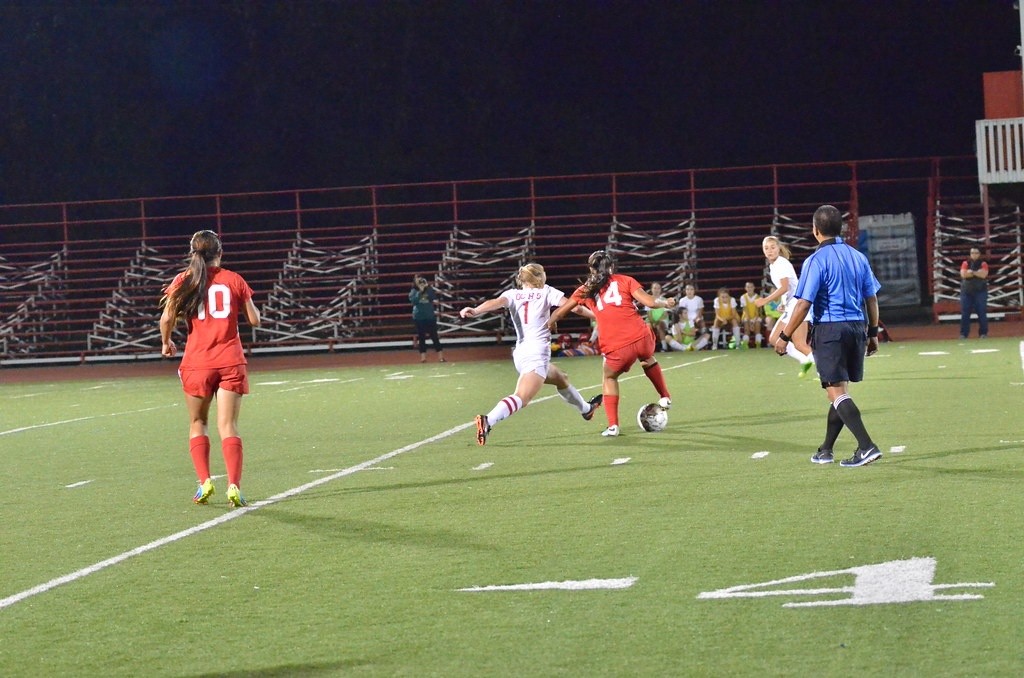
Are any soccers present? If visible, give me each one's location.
[637,403,668,432]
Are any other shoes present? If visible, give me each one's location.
[741,338,750,350]
[735,345,740,350]
[711,345,718,350]
[420,359,427,364]
[440,358,447,363]
[686,343,697,351]
[958,335,967,340]
[979,334,987,340]
[755,339,761,349]
[659,348,669,354]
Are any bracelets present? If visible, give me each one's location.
[779,331,790,341]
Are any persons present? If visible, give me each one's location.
[678,283,707,335]
[665,307,710,351]
[644,282,669,351]
[958,246,989,339]
[549,316,604,357]
[740,282,763,349]
[409,273,446,362]
[754,236,820,380]
[774,205,883,467]
[160,230,259,506]
[548,250,676,437]
[711,287,741,350]
[460,263,603,446]
[763,286,783,348]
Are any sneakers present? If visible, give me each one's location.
[192,477,218,503]
[226,483,247,508]
[798,359,814,378]
[810,445,837,464]
[601,424,622,438]
[656,396,673,409]
[580,394,604,421]
[474,414,492,446]
[840,442,882,468]
